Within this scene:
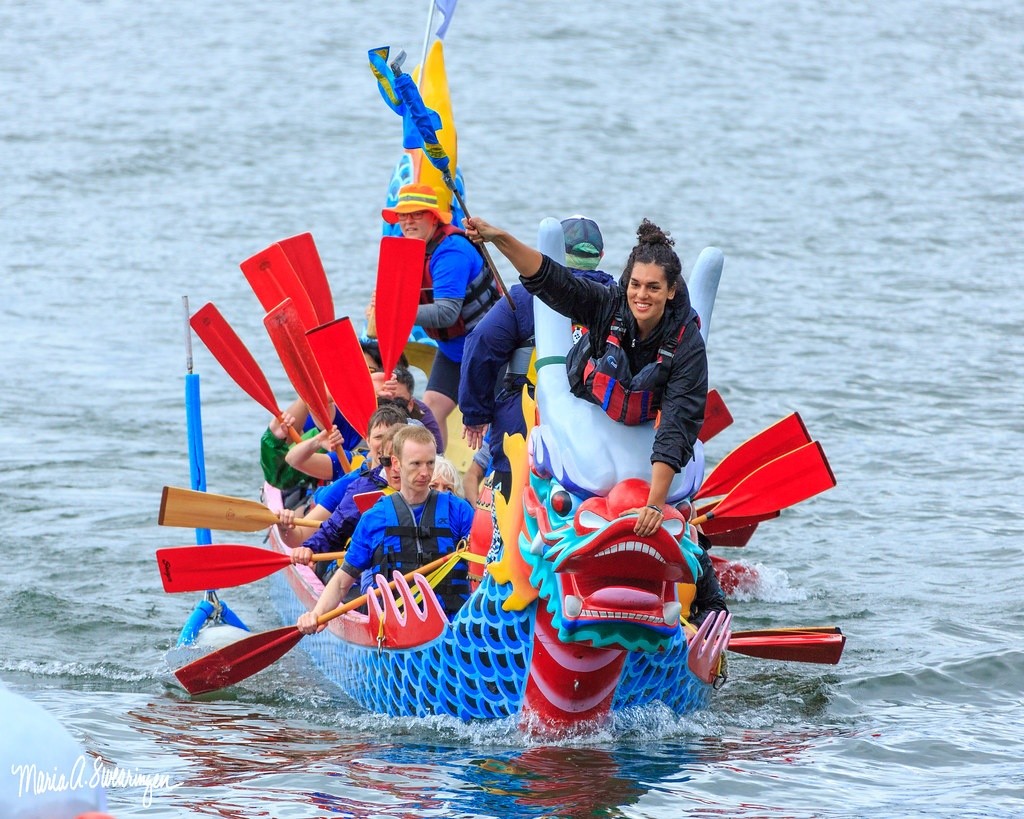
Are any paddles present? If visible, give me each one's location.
[155,230,849,696]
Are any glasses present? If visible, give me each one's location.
[395,212,431,220]
[379,455,393,467]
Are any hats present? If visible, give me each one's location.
[561,218,605,259]
[381,185,453,224]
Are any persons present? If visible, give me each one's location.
[381,184,503,457]
[462,216,710,538]
[260,342,473,635]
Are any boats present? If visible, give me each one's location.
[255,219,733,744]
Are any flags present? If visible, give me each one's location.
[368,47,449,172]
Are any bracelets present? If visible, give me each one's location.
[647,505,662,513]
